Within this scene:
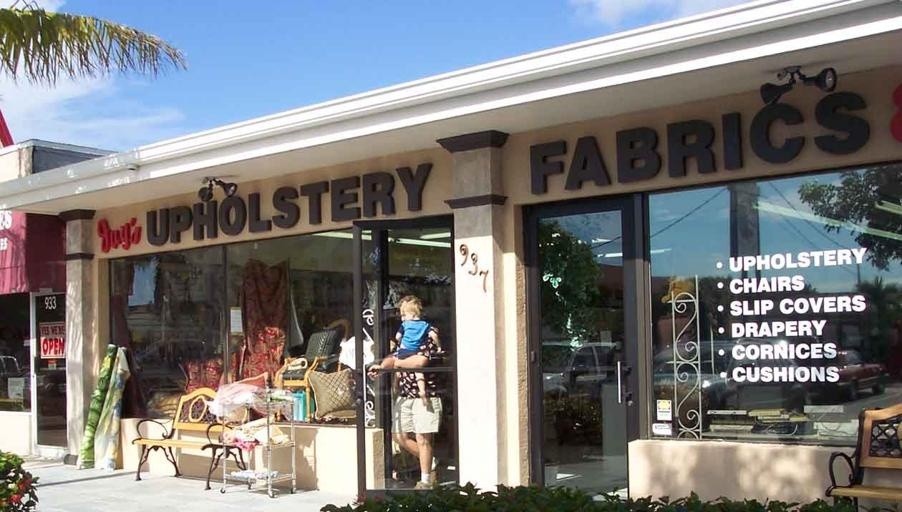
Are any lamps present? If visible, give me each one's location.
[199,176,238,202]
[761,64,837,108]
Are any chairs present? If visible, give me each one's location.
[275,319,352,423]
[827,403,902,511]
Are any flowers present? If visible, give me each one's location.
[1,451,42,512]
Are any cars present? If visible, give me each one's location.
[0,355,65,418]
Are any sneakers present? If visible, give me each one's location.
[429,456,441,489]
[412,480,438,491]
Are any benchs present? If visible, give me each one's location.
[132,387,253,494]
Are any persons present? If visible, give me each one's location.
[393,297,442,407]
[366,293,445,492]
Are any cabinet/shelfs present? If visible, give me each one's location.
[218,386,299,498]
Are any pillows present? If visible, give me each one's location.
[305,326,357,418]
